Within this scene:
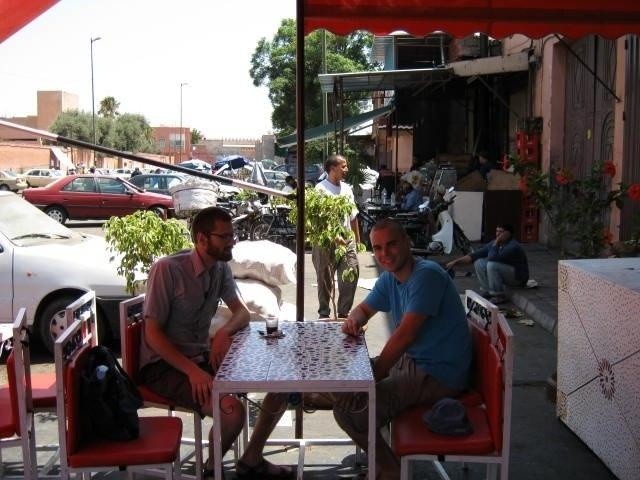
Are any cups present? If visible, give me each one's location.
[265,316,279,335]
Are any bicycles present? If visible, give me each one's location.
[225,194,296,248]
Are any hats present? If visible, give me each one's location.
[422,399,472,434]
[377,165,389,171]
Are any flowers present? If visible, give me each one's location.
[517,159,640,260]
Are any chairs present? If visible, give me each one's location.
[118,292,241,479]
[0,291,98,479]
[55,319,182,480]
[390,288,515,480]
[0,307,39,479]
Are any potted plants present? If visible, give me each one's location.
[268,182,368,408]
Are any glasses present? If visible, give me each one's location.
[209,233,237,240]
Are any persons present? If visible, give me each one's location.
[312,155,359,319]
[399,165,427,193]
[397,184,423,247]
[333,217,473,479]
[139,209,292,479]
[281,177,297,194]
[131,168,144,188]
[82,167,97,187]
[445,224,529,306]
[149,169,161,186]
[474,152,512,236]
[374,165,395,202]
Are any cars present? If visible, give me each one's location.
[16,168,72,189]
[0,170,29,191]
[0,190,160,360]
[74,162,322,225]
[19,173,175,226]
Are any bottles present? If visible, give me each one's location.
[390,191,396,207]
[381,187,388,205]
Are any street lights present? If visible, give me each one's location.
[91,33,101,150]
[180,81,190,153]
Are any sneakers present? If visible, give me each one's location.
[479,291,506,304]
[342,453,369,466]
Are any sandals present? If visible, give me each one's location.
[203,457,293,479]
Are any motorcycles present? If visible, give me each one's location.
[404,184,475,260]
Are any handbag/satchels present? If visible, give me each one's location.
[73,346,140,441]
